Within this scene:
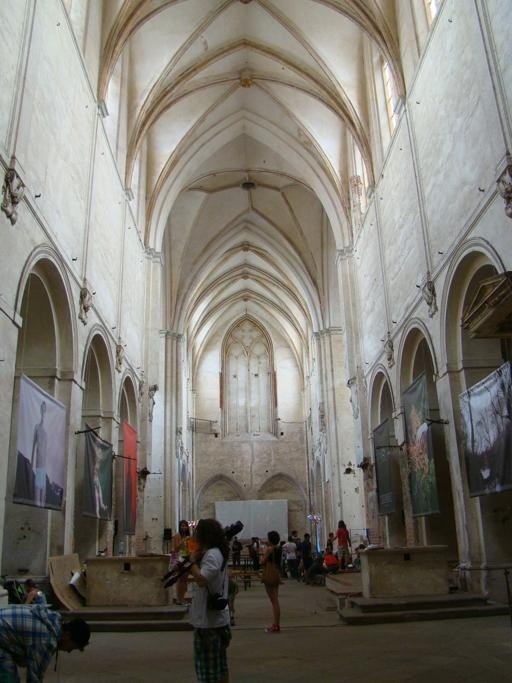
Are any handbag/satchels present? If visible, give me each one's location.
[205,585,229,611]
[260,547,281,587]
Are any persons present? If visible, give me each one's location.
[89,433,112,518]
[29,399,49,505]
[170,519,191,605]
[0,602,92,682]
[23,579,48,605]
[232,519,367,585]
[183,519,232,683]
[261,531,283,633]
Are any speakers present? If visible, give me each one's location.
[164,528,172,540]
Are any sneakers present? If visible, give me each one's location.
[173,597,191,607]
[264,623,281,633]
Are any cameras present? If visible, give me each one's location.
[207,594,228,611]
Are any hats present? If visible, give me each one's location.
[64,617,91,653]
